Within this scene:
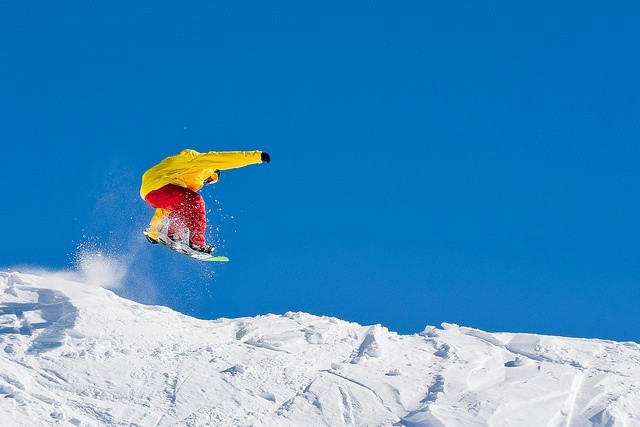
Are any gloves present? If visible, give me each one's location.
[261,152,270,163]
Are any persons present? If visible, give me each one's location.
[140,148,271,255]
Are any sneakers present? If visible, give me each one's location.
[189,239,215,254]
[167,233,188,244]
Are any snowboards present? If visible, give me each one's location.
[142,232,229,262]
